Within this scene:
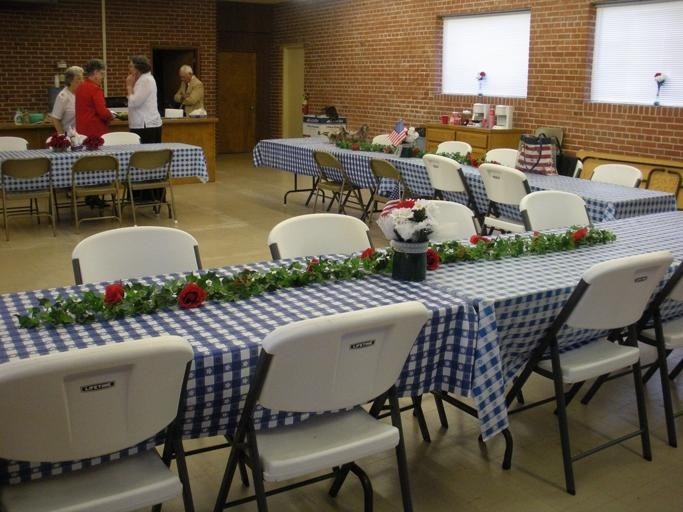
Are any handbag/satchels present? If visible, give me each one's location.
[515,133,558,175]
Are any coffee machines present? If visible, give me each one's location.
[466,103,488,128]
[491,105,513,130]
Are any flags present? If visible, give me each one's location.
[385,121,406,145]
[380,179,402,217]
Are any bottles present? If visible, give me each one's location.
[63,131,71,152]
[13,111,23,125]
[488,105,495,130]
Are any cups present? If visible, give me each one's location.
[440,115,448,125]
[462,110,471,126]
[450,112,461,124]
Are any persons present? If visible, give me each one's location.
[75,59,115,210]
[124,55,163,202]
[51,66,84,135]
[173,65,203,116]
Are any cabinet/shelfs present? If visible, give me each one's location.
[424,122,533,155]
[0,117,219,201]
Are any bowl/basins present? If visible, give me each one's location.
[112,112,127,120]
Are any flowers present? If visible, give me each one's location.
[476,71,487,96]
[654,72,665,105]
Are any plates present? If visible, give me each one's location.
[188,115,207,118]
